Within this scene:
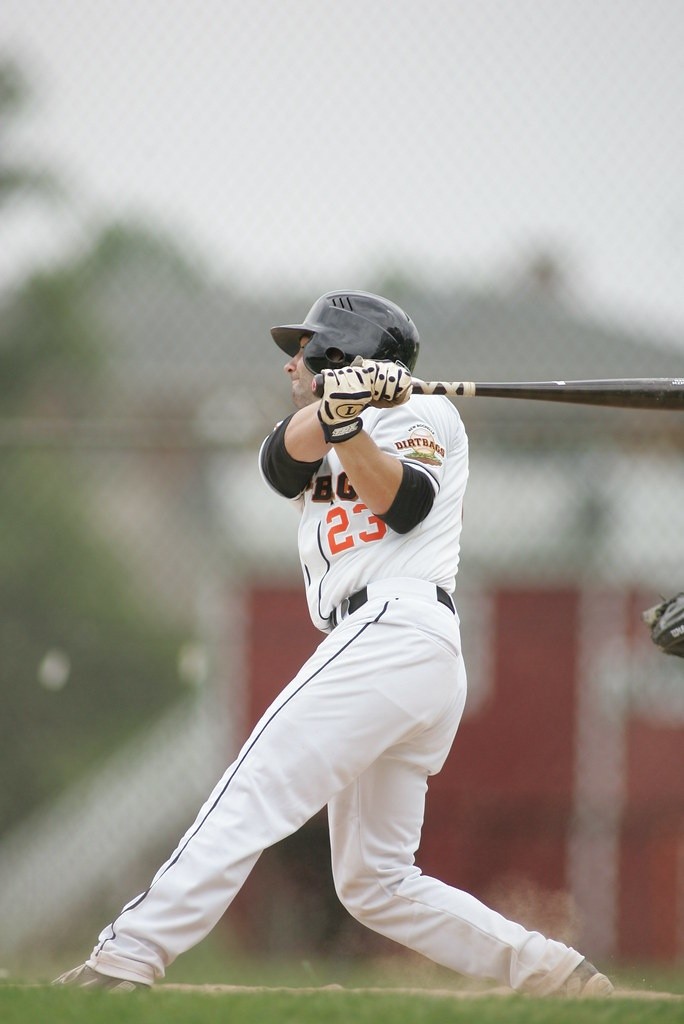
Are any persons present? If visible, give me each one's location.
[54,289,616,998]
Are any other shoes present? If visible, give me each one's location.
[537,959,612,1001]
[51,965,150,995]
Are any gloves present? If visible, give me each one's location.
[311,367,375,443]
[363,362,416,408]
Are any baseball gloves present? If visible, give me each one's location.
[640,592,684,658]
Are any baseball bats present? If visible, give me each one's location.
[312,374,683,412]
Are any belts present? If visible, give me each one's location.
[329,585,456,629]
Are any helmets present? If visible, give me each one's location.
[271,290,419,375]
